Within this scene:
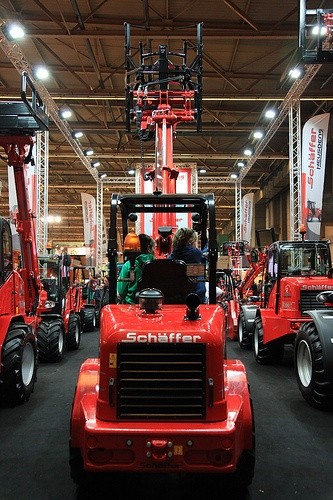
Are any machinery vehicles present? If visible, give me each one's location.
[0,0,333,487]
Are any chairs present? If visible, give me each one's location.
[138,259,195,305]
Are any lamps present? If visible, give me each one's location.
[71,132,83,139]
[90,161,100,167]
[83,149,94,156]
[244,145,254,155]
[266,109,279,117]
[237,159,247,166]
[231,173,239,178]
[292,68,305,79]
[58,110,72,119]
[98,173,107,178]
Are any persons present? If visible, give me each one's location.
[100,274,110,307]
[117,233,158,305]
[166,226,208,305]
